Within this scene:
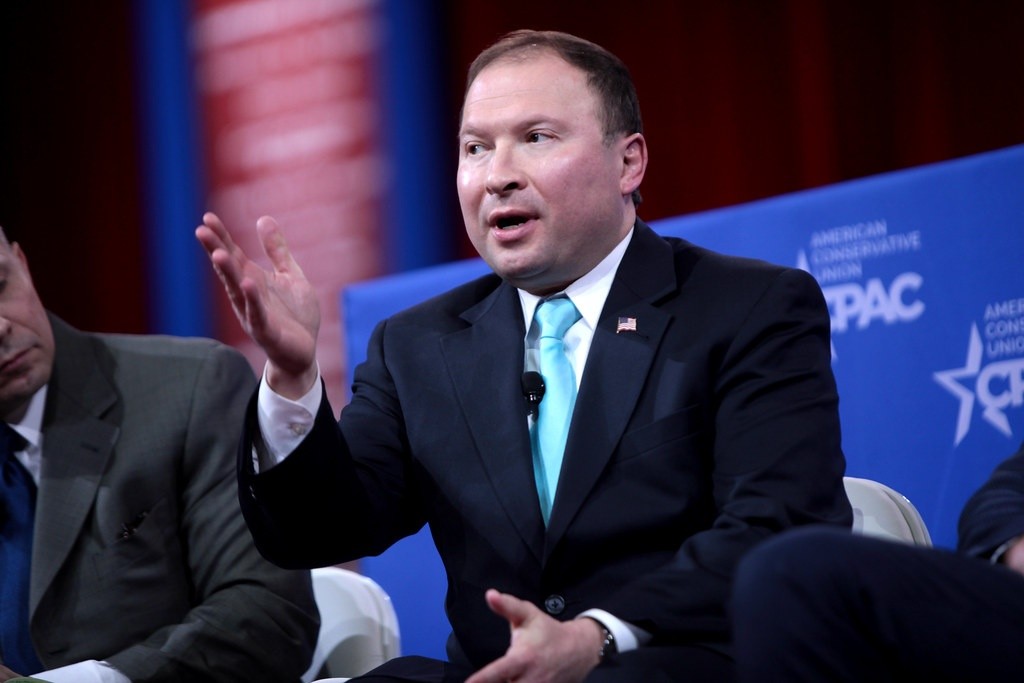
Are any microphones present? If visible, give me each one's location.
[521,371,545,407]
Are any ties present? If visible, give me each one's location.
[528,297,581,523]
[0,420,40,679]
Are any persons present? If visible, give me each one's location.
[1,226,322,683]
[729,444,1024,683]
[194,27,868,683]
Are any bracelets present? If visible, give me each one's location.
[583,612,619,666]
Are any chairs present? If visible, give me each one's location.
[842,476,934,548]
[301,565,401,683]
